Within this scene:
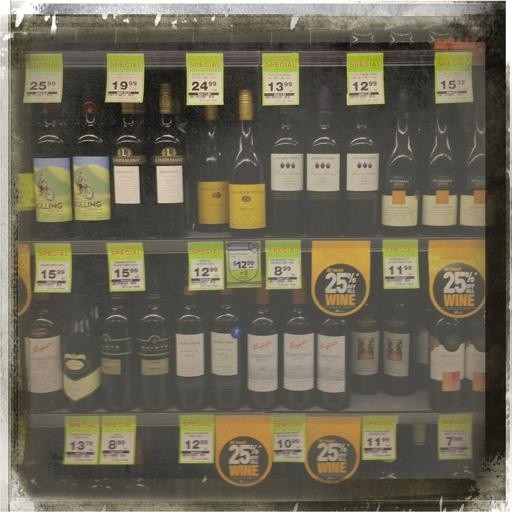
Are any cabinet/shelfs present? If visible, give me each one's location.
[6,48,508,511]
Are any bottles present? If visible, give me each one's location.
[31,80,498,237]
[23,258,498,411]
